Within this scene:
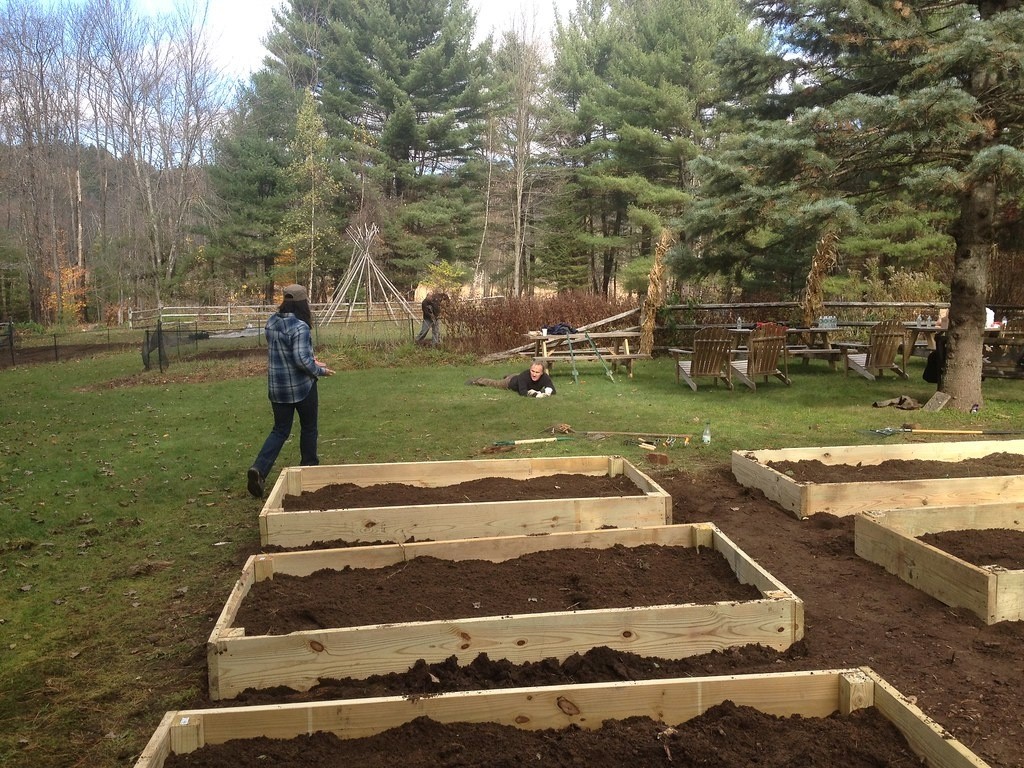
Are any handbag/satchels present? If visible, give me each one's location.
[923,351,939,383]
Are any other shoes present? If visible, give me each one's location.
[247,467,265,496]
[464,377,483,386]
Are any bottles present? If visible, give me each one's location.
[702,421,711,446]
[737,317,741,330]
[818,316,837,328]
[927,316,932,328]
[917,315,922,328]
[1001,316,1007,328]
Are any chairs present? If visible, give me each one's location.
[840,319,910,381]
[668,326,734,391]
[724,322,791,390]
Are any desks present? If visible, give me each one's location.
[911,325,1000,355]
[788,328,844,371]
[523,331,642,378]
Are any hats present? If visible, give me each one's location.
[282,283,308,302]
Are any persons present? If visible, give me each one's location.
[465,362,555,398]
[416,292,441,349]
[248,284,336,497]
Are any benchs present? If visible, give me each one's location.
[740,342,934,360]
[520,347,652,360]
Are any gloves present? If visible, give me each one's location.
[527,389,548,398]
[542,387,553,397]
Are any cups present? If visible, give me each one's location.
[542,329,548,336]
[545,388,553,397]
